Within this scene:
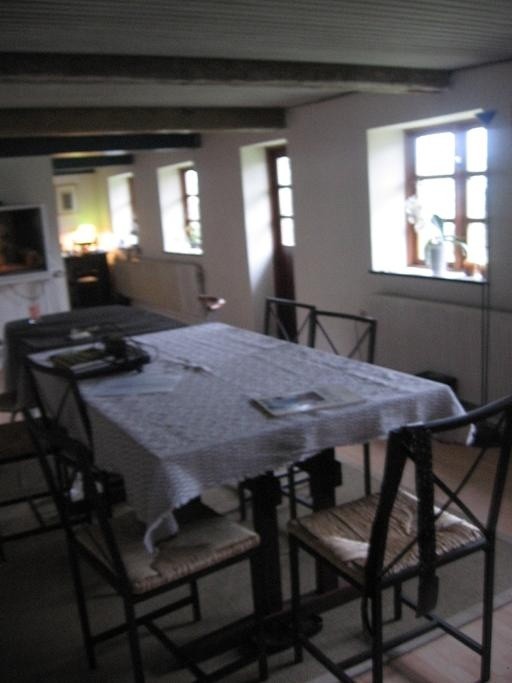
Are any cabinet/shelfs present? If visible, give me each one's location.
[61,251,114,310]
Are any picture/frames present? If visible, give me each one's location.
[54,183,78,214]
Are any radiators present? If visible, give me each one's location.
[137,257,206,318]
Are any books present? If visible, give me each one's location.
[250,382,365,418]
[49,346,114,372]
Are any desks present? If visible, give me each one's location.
[7,299,465,661]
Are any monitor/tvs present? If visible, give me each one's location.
[0,203,50,286]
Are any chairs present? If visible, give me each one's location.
[0,379,85,536]
[11,348,267,683]
[264,300,313,355]
[293,392,511,680]
[307,308,379,498]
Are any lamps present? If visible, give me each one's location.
[73,223,97,255]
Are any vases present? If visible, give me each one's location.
[431,241,447,275]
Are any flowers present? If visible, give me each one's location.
[405,195,468,268]
